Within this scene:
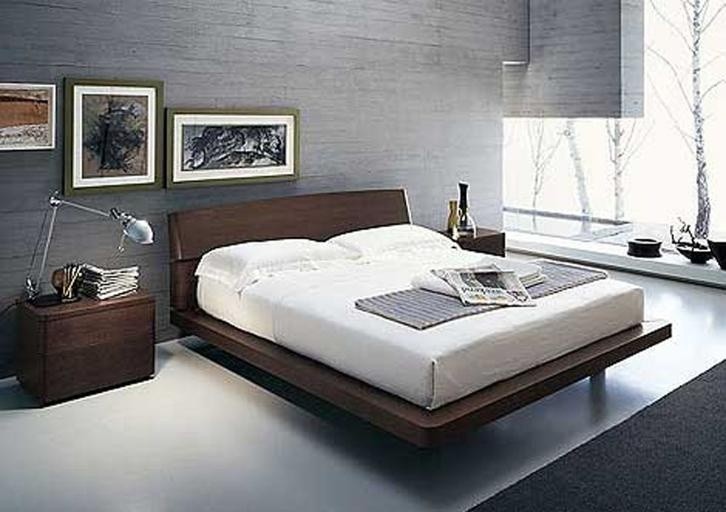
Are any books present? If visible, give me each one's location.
[430,263,537,307]
[82,263,140,301]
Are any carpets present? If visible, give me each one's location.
[462,356,726,512]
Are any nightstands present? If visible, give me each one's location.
[14,289,157,408]
[438,228,505,257]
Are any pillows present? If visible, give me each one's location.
[194,238,361,293]
[324,223,461,263]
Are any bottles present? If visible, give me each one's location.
[448,183,477,240]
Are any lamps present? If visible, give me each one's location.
[23,189,154,307]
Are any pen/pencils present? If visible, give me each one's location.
[61,263,83,298]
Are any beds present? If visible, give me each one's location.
[167,188,672,449]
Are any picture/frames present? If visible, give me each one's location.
[165,108,300,189]
[0,83,57,151]
[62,76,164,197]
